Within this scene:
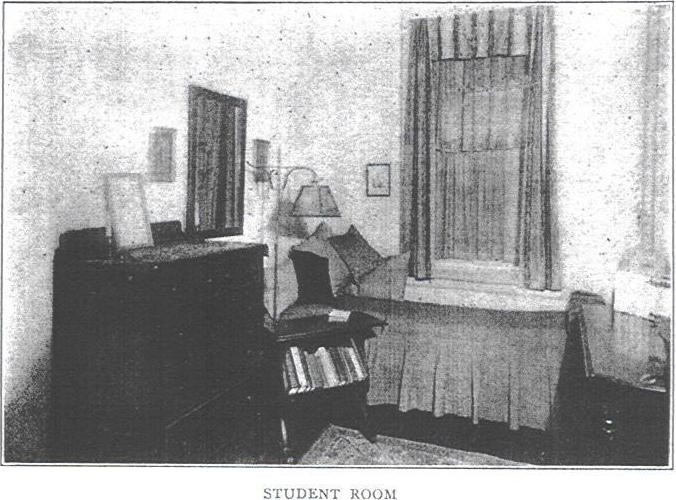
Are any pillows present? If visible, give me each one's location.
[260,259,302,316]
[287,223,349,294]
[329,227,384,279]
[341,250,412,302]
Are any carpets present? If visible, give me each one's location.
[297,423,534,465]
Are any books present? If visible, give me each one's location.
[281,342,368,396]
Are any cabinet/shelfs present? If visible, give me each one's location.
[262,303,389,463]
[52,239,270,456]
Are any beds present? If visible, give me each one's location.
[261,225,612,434]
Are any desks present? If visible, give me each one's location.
[572,272,670,465]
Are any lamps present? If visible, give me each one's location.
[273,165,342,324]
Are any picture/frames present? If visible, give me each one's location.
[254,140,272,181]
[105,170,154,251]
[363,163,392,199]
[150,126,176,184]
